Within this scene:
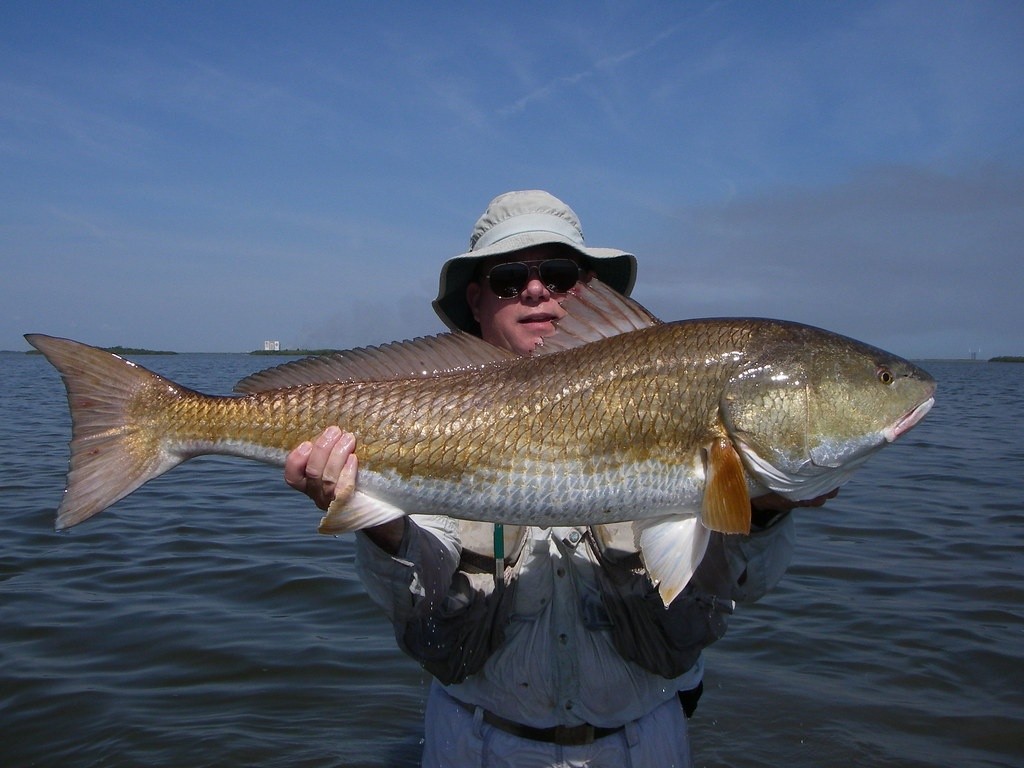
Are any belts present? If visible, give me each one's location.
[451,695,626,746]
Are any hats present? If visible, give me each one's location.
[431,189,639,331]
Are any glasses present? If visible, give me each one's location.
[485,258,582,300]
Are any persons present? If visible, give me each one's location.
[283,190,839,767]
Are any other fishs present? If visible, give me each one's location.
[22,277,937,533]
